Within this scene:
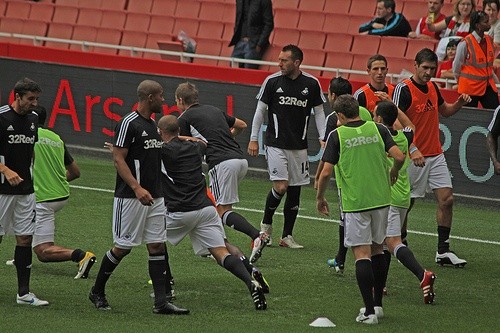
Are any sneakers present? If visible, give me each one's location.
[359,306,384,318]
[355,312,379,324]
[419,271,438,304]
[383,289,387,294]
[16,292,49,306]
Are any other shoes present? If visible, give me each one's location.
[89,287,112,311]
[435,250,467,266]
[253,270,270,293]
[148,278,175,285]
[265,231,272,246]
[250,231,270,263]
[74,251,97,281]
[153,302,190,315]
[327,258,345,273]
[5,259,15,266]
[251,286,267,310]
[279,234,303,248]
[150,291,176,299]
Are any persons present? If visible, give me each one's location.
[0,77,50,306]
[227,0,274,69]
[392,49,471,268]
[88,80,270,315]
[6,106,97,279]
[313,54,438,325]
[247,44,327,249]
[359,0,412,37]
[408,0,500,109]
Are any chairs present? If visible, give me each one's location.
[0,0,500,107]
